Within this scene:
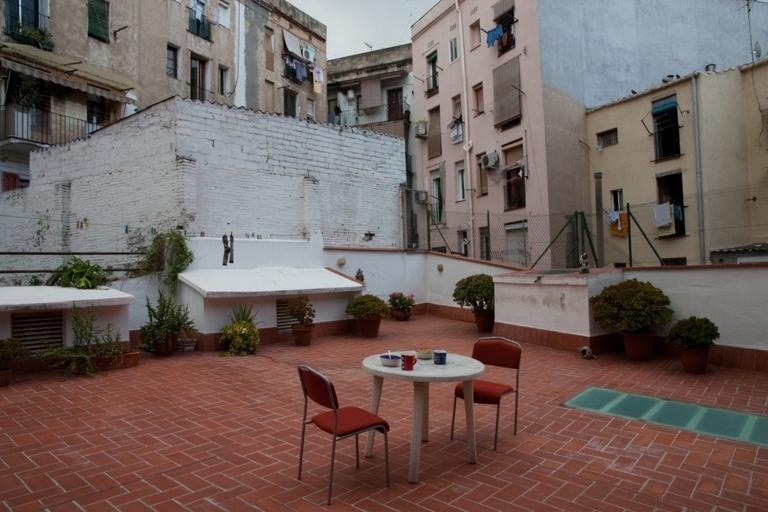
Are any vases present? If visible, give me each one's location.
[393,308,411,319]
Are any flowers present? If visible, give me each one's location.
[387,289,413,308]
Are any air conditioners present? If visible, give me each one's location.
[481,150,501,170]
[301,45,316,65]
[415,120,431,140]
[412,190,427,207]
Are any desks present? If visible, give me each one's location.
[360,344,488,485]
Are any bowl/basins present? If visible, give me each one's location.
[415,348,433,359]
[380,354,401,366]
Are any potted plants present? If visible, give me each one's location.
[345,293,385,340]
[453,273,495,335]
[283,293,318,348]
[139,286,199,358]
[673,310,721,374]
[1,334,29,390]
[586,272,674,362]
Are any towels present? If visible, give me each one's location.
[653,203,683,237]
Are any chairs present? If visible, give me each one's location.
[290,360,397,506]
[449,335,525,450]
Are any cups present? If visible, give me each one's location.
[401,351,418,370]
[433,349,446,363]
